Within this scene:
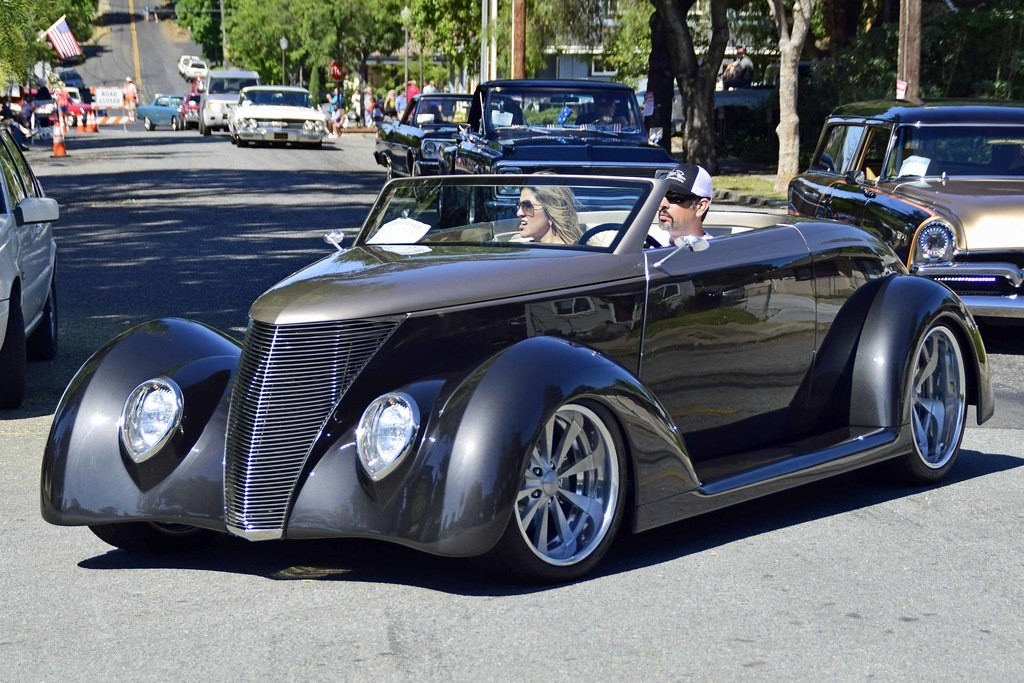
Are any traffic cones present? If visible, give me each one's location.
[51,122,70,158]
[59,109,100,133]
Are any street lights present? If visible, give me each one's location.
[280,34,288,86]
[400,7,412,106]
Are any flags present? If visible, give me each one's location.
[46,19,84,58]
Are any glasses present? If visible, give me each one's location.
[664,189,705,203]
[515,200,544,217]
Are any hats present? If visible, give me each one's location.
[664,165,713,200]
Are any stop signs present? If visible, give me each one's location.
[329,60,347,80]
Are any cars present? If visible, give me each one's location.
[136,95,185,131]
[627,58,851,145]
[440,81,680,231]
[4,67,85,119]
[374,91,526,202]
[40,175,998,578]
[178,93,202,129]
[178,56,207,94]
[227,86,327,148]
[0,125,60,398]
[786,98,1023,327]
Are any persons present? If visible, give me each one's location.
[517,171,586,245]
[657,163,713,245]
[143,4,158,22]
[591,97,629,125]
[321,77,456,140]
[1,76,139,150]
[721,44,754,90]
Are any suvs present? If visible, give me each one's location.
[198,71,261,135]
